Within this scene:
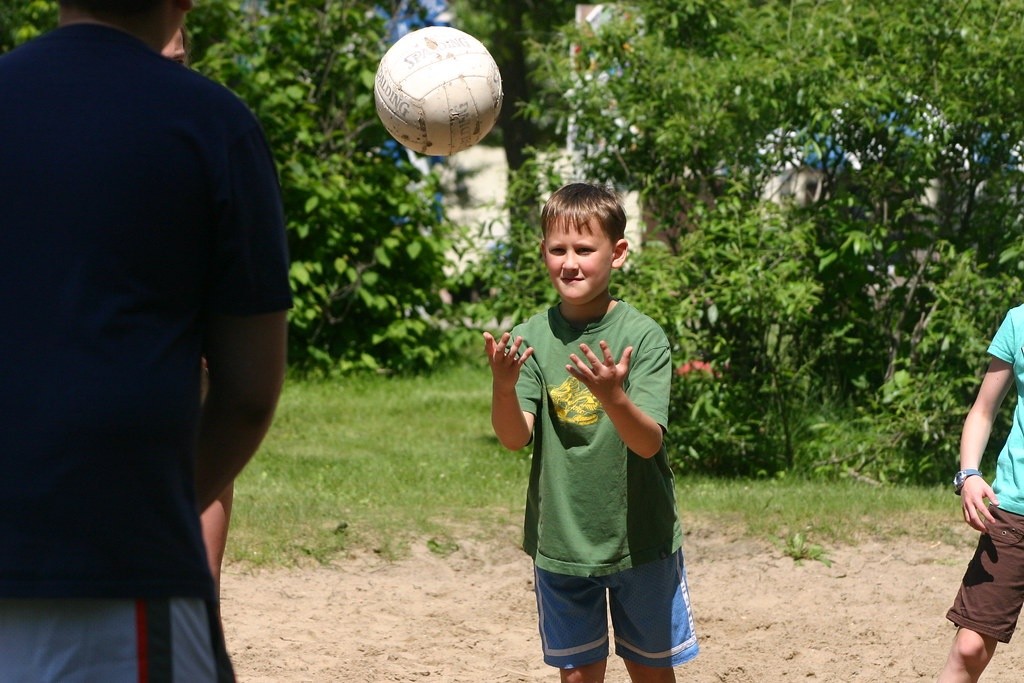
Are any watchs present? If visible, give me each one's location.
[953,469,984,496]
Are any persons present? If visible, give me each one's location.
[936,306,1024,683]
[478,184,699,683]
[0,0,296,683]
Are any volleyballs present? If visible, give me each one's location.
[373,25,504,157]
